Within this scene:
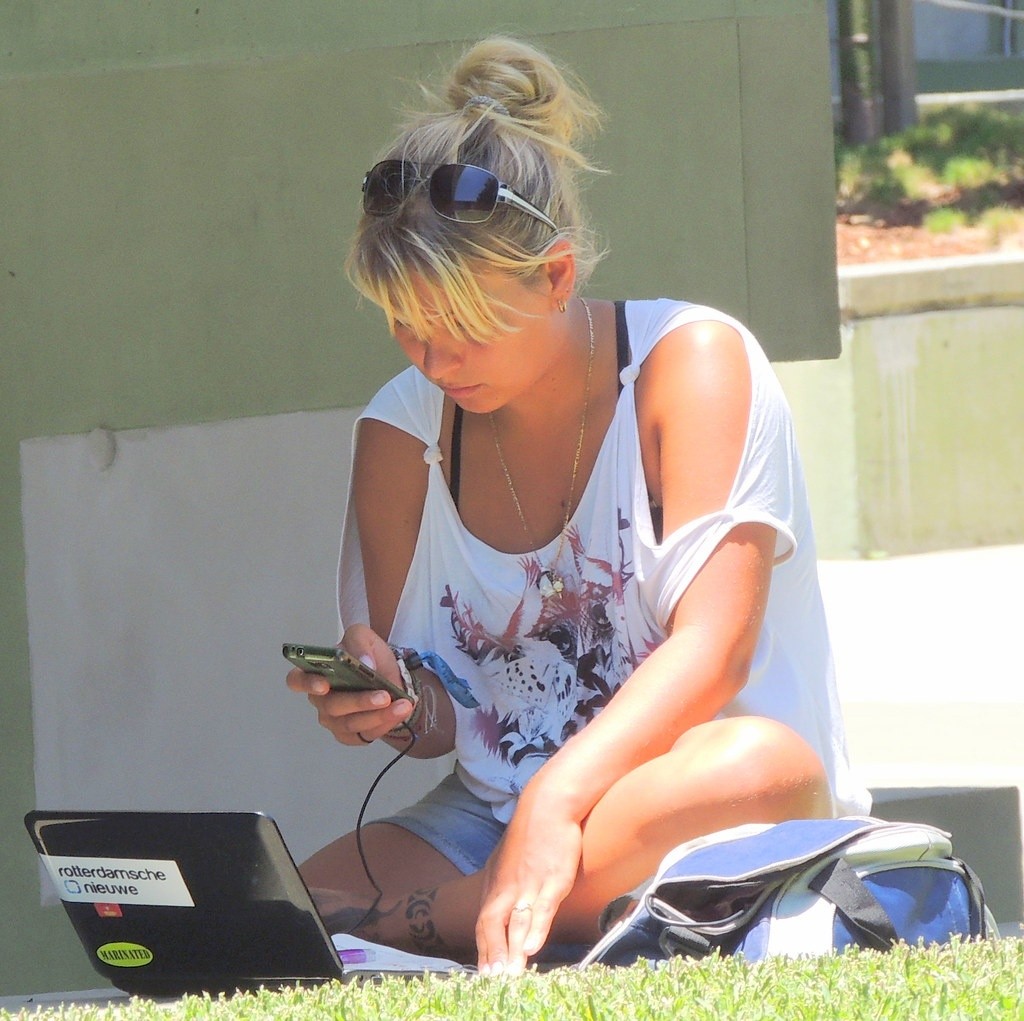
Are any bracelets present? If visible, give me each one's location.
[385,646,423,739]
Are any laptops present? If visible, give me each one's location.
[23,810,480,997]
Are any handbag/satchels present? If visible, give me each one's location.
[577,814,1002,966]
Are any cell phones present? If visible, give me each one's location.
[280,643,415,706]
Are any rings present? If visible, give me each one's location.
[358,732,373,744]
[513,900,532,911]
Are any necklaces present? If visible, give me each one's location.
[489,295,594,600]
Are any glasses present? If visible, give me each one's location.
[362,161,562,242]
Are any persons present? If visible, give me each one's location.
[300,36,872,980]
